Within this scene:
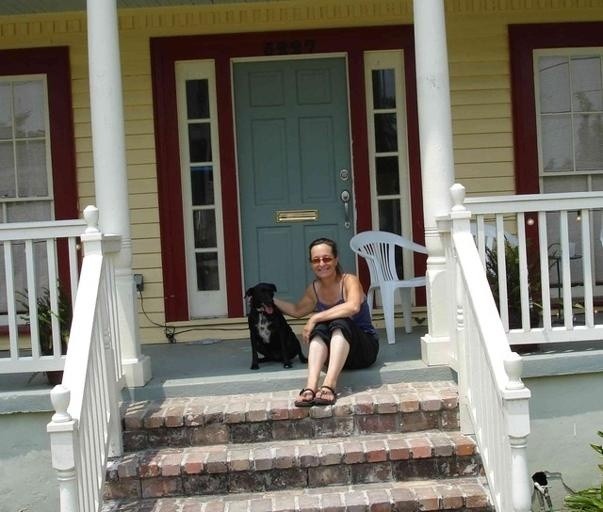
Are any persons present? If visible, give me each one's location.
[248,238,379,407]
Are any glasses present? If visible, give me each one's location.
[311,256,335,265]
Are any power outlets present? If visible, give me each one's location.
[132,274,143,294]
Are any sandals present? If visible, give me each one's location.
[314,386,337,405]
[294,387,316,407]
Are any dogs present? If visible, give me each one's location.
[244,282,308,370]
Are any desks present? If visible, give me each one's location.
[543,250,585,326]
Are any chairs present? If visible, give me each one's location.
[349,229,428,344]
[468,223,519,276]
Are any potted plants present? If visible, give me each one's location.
[482,235,560,354]
[13,279,72,387]
